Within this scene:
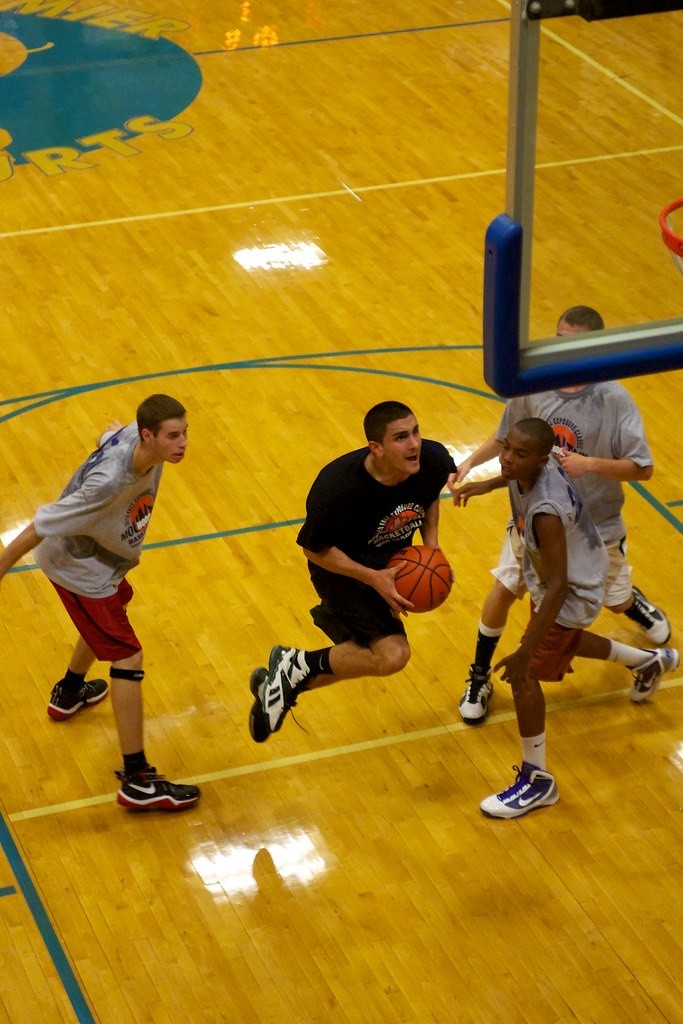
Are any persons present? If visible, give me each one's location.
[249,400,457,743]
[0,393,201,812]
[447,306,671,727]
[454,418,677,819]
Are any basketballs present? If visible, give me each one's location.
[385,545,454,614]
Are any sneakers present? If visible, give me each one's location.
[47,678,109,721]
[480,761,559,819]
[112,764,201,812]
[249,667,297,743]
[459,664,494,720]
[625,646,679,702]
[263,645,316,733]
[623,584,671,645]
